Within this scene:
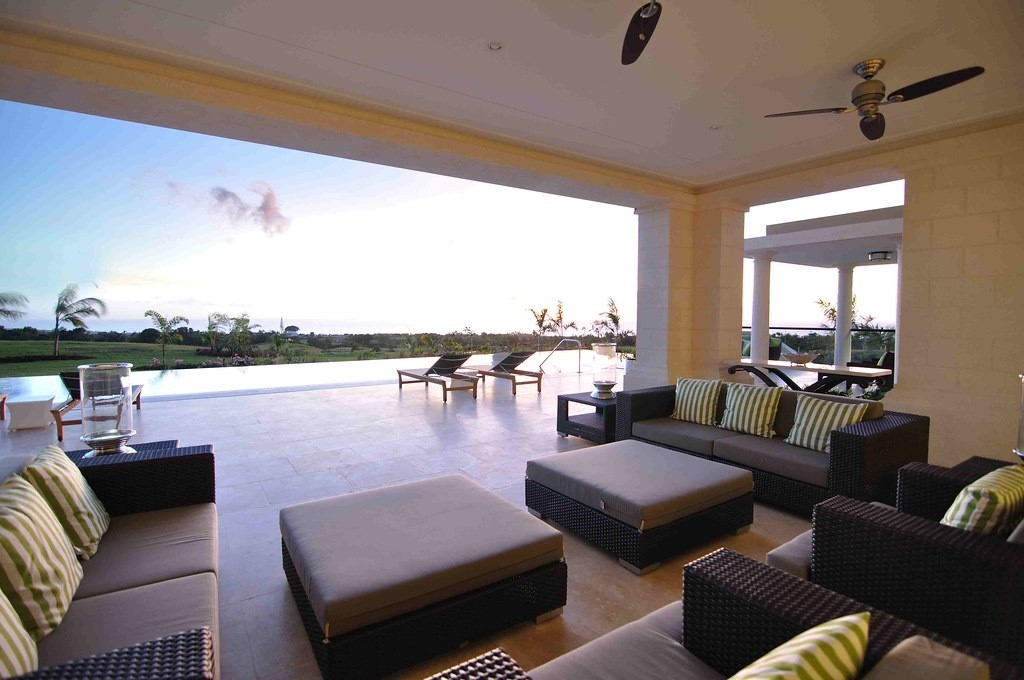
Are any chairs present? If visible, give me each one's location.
[464,350,544,394]
[423,547,1023,679]
[845,351,895,392]
[52,373,144,440]
[767,462,1024,656]
[397,352,479,403]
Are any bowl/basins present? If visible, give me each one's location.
[780,352,820,364]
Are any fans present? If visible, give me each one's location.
[762,60,984,141]
[622,0,663,67]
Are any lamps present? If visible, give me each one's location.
[868,251,893,262]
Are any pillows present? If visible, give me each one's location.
[726,612,871,679]
[787,393,867,455]
[674,377,721,427]
[941,465,1024,535]
[0,446,110,676]
[721,384,782,439]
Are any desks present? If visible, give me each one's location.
[6,394,54,433]
[722,357,893,392]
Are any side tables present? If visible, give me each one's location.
[557,390,621,443]
[954,455,1017,474]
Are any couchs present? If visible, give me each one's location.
[9,444,222,680]
[614,385,931,516]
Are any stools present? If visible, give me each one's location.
[524,438,757,573]
[279,473,568,678]
